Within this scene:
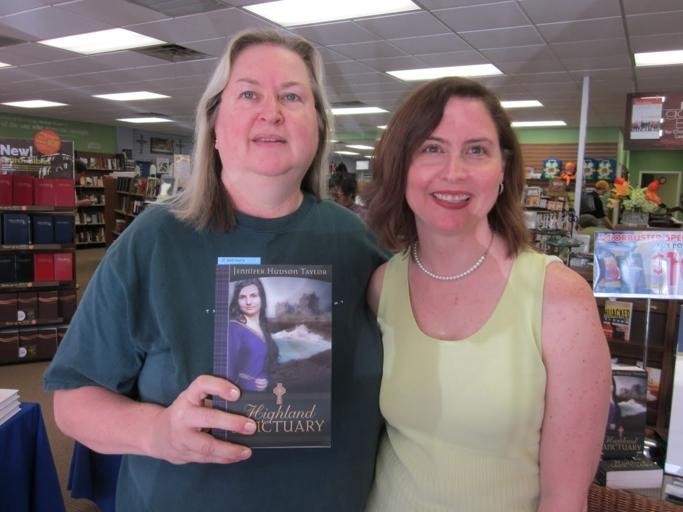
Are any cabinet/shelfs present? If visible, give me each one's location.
[523,176,614,257]
[0,153,158,365]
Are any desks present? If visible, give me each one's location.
[0,402,66,512]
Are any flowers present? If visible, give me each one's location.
[596,177,661,212]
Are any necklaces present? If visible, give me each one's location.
[413,224,495,280]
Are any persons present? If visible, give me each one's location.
[227,278,280,393]
[358,78,612,512]
[329,173,369,222]
[44,30,397,512]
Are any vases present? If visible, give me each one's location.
[621,209,650,225]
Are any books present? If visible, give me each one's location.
[0,154,176,363]
[520,178,682,505]
[214,264,333,448]
